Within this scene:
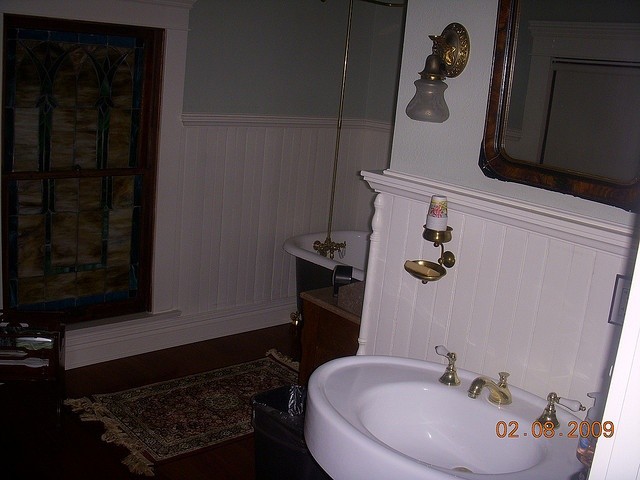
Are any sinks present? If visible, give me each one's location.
[284,229,367,322]
[304,355,586,480]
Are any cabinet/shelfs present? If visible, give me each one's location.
[297,280,365,384]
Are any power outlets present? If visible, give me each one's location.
[607,273,631,327]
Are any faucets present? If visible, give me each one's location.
[469,373,511,406]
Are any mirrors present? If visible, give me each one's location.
[477,1,640,217]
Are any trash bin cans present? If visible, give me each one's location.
[250,384,320,479]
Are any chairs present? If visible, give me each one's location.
[5,319,66,387]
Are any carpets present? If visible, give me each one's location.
[62,348,299,477]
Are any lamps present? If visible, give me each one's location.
[404,20,471,124]
